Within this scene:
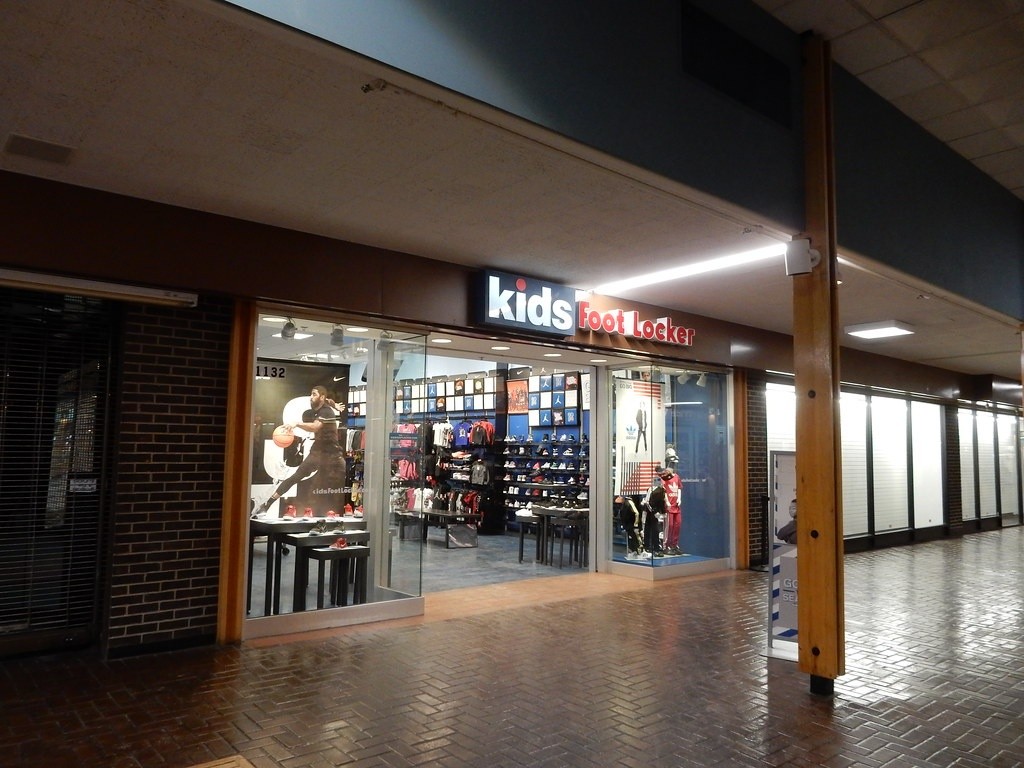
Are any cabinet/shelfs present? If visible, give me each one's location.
[504,441,589,539]
[449,450,479,483]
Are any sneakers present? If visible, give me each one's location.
[354,506,363,517]
[627,549,643,561]
[503,433,589,529]
[665,547,682,555]
[302,507,313,521]
[344,504,353,516]
[329,537,348,549]
[654,551,664,557]
[332,521,346,533]
[283,504,297,520]
[638,550,652,559]
[676,545,691,557]
[308,519,328,535]
[327,511,336,521]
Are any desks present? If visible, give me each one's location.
[514,482,590,571]
[246,517,370,617]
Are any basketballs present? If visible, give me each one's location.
[273,426,295,447]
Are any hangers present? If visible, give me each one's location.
[388,417,491,494]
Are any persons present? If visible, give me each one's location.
[634,399,649,453]
[645,478,670,558]
[776,499,797,547]
[262,385,347,519]
[616,496,643,559]
[661,468,684,555]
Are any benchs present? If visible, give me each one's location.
[394,508,482,550]
